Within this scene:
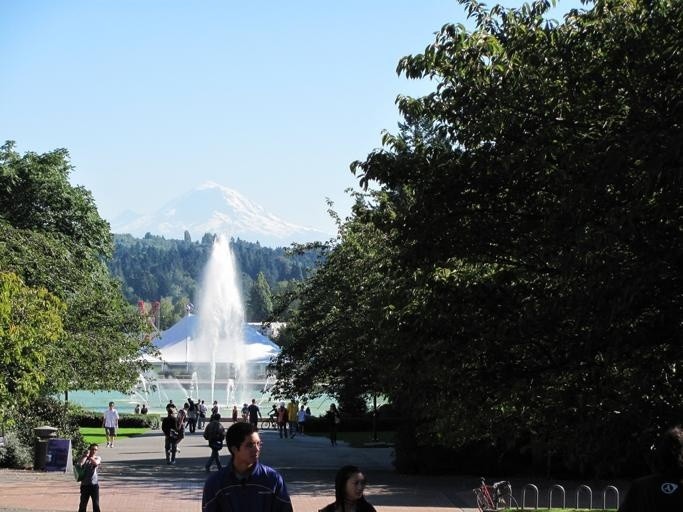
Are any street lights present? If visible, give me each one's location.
[186,302,193,317]
[185,335,191,373]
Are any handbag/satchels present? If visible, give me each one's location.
[334,410,340,424]
[170,428,179,439]
[74,464,85,482]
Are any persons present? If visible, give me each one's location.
[102,401,119,447]
[318,465,376,512]
[200,421,292,511]
[321,402,339,447]
[202,413,226,471]
[134,404,140,414]
[78,444,102,512]
[617,425,683,511]
[159,398,310,465]
[140,404,148,415]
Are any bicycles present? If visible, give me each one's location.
[473,476,519,511]
[260,412,279,430]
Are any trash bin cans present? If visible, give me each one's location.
[33,426,58,469]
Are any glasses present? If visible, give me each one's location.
[244,442,264,449]
